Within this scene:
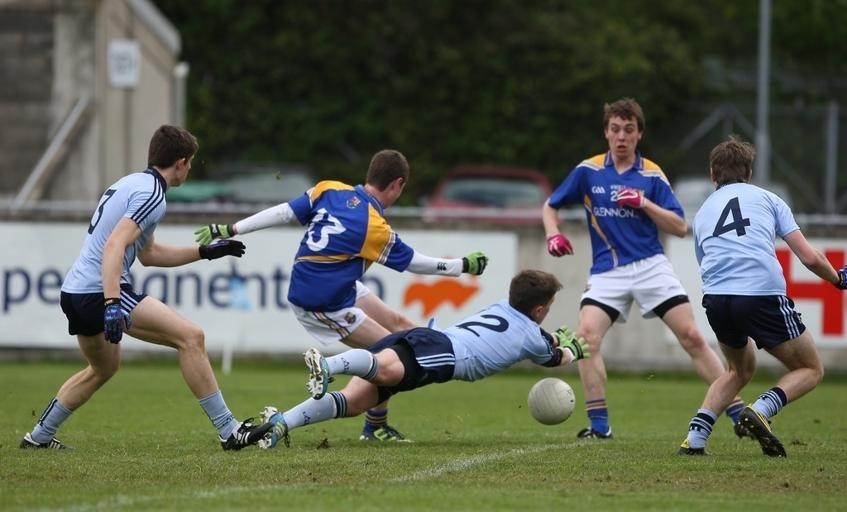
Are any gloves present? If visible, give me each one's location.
[616,188,647,211]
[103,295,133,346]
[193,222,236,247]
[546,233,576,258]
[461,251,489,277]
[550,325,593,364]
[835,264,847,291]
[197,239,247,262]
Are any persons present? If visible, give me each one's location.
[258,269,589,449]
[194,150,490,443]
[21,125,274,452]
[677,135,847,457]
[540,97,753,440]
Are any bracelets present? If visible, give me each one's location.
[105,298,120,304]
[643,196,648,208]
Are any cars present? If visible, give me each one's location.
[670,179,794,229]
[418,166,558,228]
[206,164,316,222]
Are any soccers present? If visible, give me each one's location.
[528,378,576,426]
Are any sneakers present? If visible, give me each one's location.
[218,418,275,453]
[575,424,615,440]
[675,437,718,457]
[740,403,788,459]
[734,419,753,440]
[256,405,289,451]
[302,346,335,401]
[357,422,418,444]
[18,430,77,452]
